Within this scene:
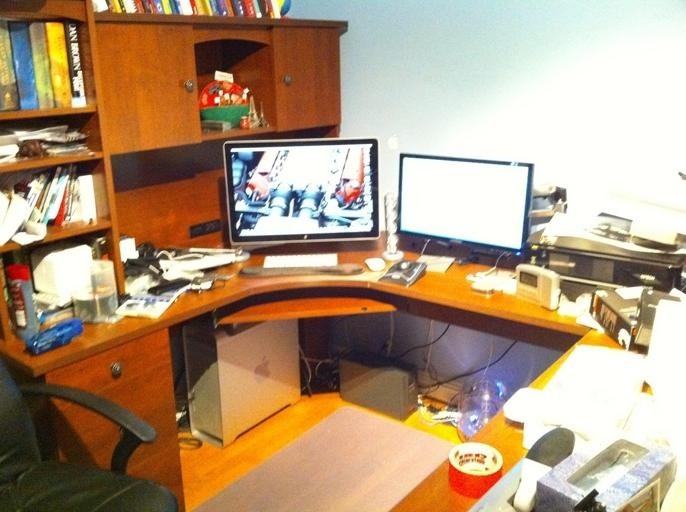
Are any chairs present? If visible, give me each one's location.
[0,360,179,512]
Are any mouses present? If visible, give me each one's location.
[365,258,386,272]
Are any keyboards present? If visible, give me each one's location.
[263,253,338,268]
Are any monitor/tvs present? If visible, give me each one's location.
[223,138,381,276]
[397,154,534,262]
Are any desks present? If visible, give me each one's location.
[0,233,686,512]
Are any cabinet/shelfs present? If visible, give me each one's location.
[0,0,126,340]
[93,11,349,299]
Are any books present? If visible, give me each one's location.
[0,19,86,112]
[0,125,98,251]
[92,0,283,19]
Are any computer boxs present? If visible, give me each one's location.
[339,347,418,422]
[181,320,301,449]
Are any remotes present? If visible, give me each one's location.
[148,279,191,295]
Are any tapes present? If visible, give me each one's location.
[448,442,504,500]
